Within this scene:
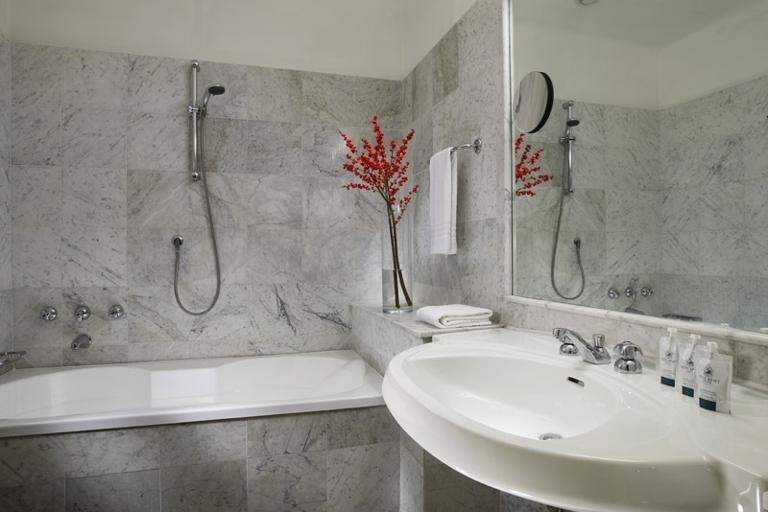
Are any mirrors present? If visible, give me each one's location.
[500,0,768,346]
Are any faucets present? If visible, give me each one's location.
[552,326,614,364]
[0,349,27,369]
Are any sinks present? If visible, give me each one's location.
[382,326,767,510]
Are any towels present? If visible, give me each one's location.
[426,146,459,256]
[414,304,493,329]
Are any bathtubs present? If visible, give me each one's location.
[0,347,387,439]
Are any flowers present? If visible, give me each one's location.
[337,113,421,308]
[514,132,555,198]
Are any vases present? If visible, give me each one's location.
[380,213,414,314]
